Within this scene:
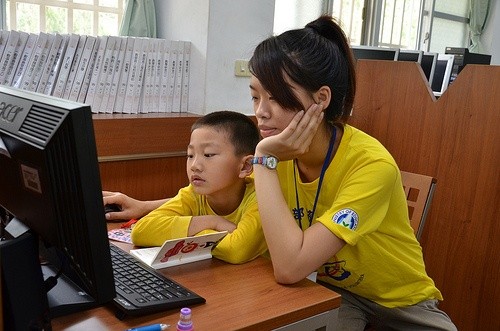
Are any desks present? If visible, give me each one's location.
[0,220,343,331]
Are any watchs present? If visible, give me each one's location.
[252,156,278,169]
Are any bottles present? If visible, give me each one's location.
[176,307,194,331]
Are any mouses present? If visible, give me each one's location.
[103,203,124,223]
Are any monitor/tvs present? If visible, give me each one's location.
[0,83,117,331]
[348,45,455,98]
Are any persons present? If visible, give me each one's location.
[132,111,271,265]
[102,15,458,331]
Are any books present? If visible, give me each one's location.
[130,231,228,270]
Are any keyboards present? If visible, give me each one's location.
[107,242,207,320]
